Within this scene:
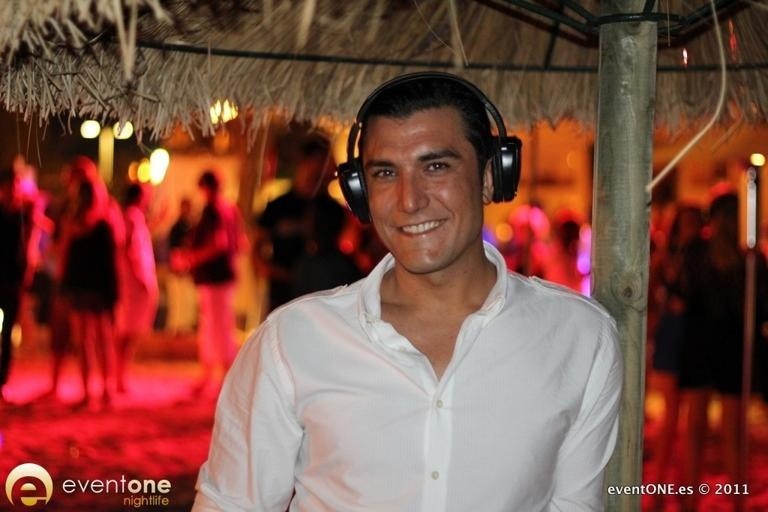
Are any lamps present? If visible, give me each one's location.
[111,120,136,140]
[79,118,102,141]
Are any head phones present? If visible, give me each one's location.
[336,70,521,222]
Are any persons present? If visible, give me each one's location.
[2,73,768,510]
